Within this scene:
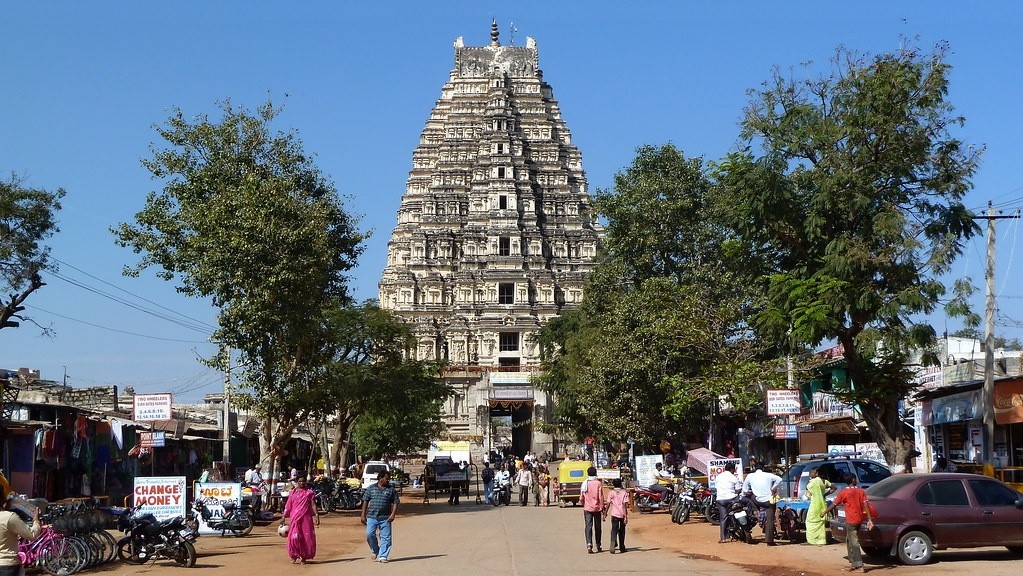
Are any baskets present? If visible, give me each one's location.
[52,511,106,532]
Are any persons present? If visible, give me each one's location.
[579,467,604,554]
[714,463,741,543]
[280,474,319,567]
[360,470,399,563]
[243,464,262,511]
[0,474,39,576]
[480,449,559,508]
[648,462,672,506]
[448,462,461,504]
[803,467,837,546]
[603,479,629,554]
[460,461,472,495]
[286,463,298,488]
[818,473,873,574]
[742,462,782,546]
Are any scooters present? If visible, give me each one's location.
[669,477,759,544]
[186,489,257,536]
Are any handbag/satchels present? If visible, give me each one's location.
[483,475,492,485]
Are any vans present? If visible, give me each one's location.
[360,460,390,489]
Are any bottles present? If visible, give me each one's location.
[11,496,37,518]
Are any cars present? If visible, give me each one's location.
[777,451,896,530]
[829,472,1023,565]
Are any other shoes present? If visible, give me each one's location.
[659,502,668,506]
[290,559,295,563]
[621,549,628,553]
[767,541,776,546]
[372,555,378,560]
[587,545,593,554]
[454,500,459,504]
[299,559,306,565]
[597,544,601,551]
[718,539,726,542]
[381,558,389,563]
[610,542,616,554]
[448,500,453,505]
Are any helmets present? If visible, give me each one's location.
[278,521,290,538]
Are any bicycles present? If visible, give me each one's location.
[17,497,118,576]
[306,476,365,515]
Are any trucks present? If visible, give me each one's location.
[433,455,467,493]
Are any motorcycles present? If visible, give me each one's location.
[632,480,678,513]
[557,461,592,505]
[186,509,200,532]
[118,500,197,568]
